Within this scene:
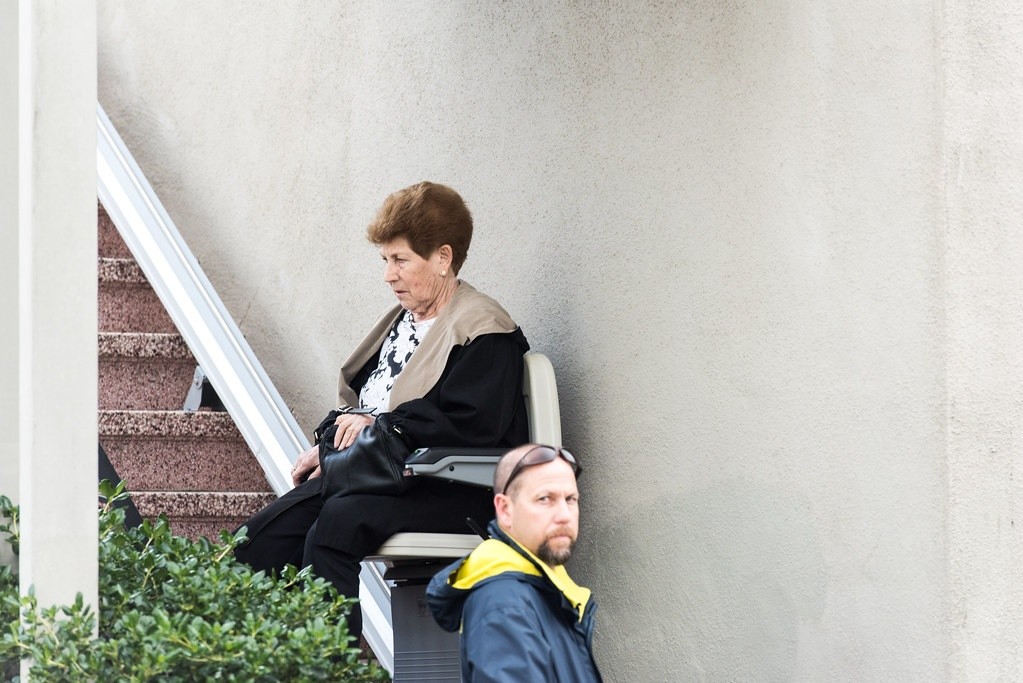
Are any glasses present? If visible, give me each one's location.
[502,446,583,498]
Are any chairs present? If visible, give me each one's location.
[360,353,565,559]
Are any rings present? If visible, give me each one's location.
[292,465,296,471]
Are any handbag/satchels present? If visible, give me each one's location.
[313,405,413,499]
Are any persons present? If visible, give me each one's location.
[231,182,530,648]
[428,445,603,683]
